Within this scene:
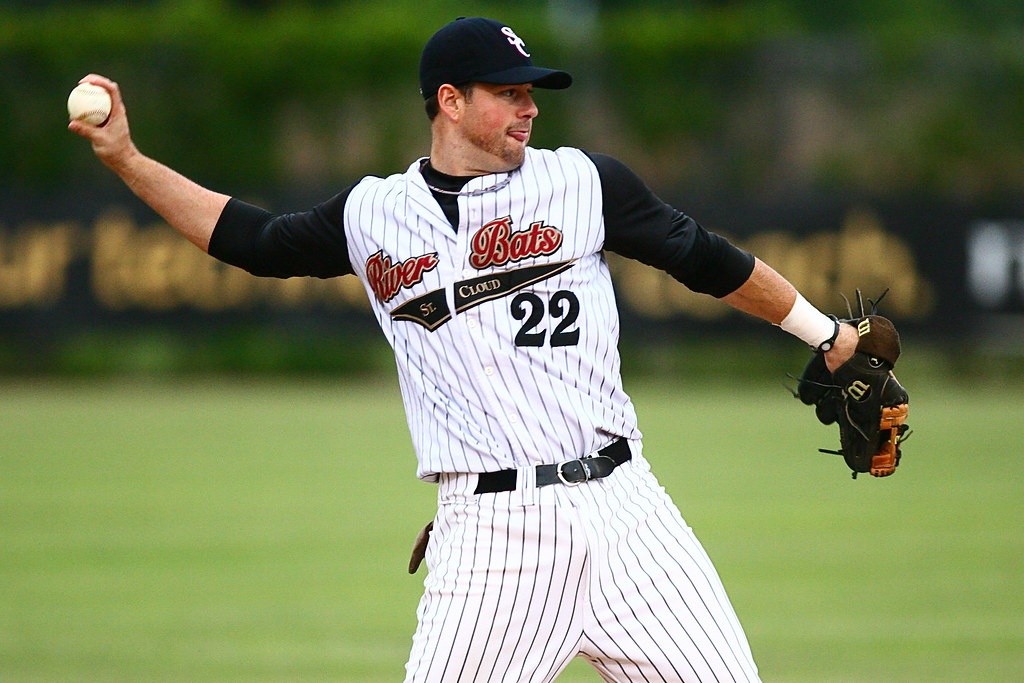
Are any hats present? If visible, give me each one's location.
[420,16,572,100]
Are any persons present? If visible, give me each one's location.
[70,14,908,683]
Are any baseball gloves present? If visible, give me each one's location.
[794,315,911,478]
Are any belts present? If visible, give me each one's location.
[474,438,632,495]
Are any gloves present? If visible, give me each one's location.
[408,520,436,573]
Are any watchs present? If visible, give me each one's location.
[809,313,840,352]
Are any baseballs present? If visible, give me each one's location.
[67,83,111,127]
